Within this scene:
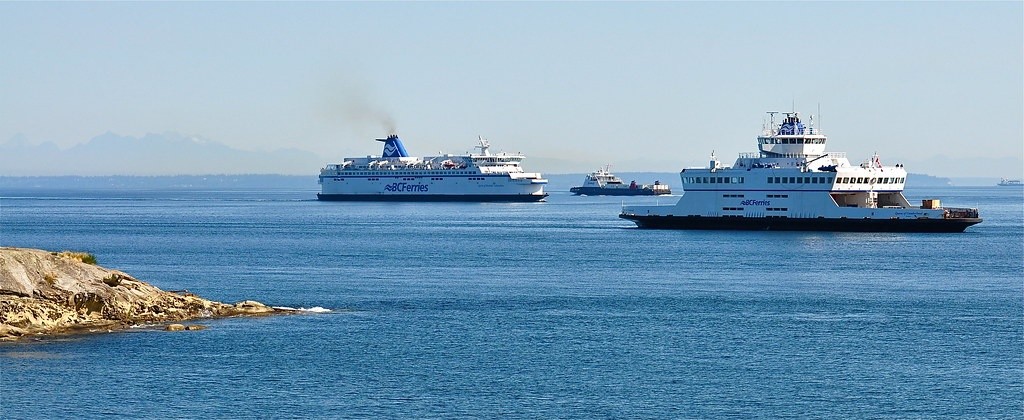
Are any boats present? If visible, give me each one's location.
[317,134,550,202]
[569,165,672,196]
[997,180,1024,187]
[619,111,983,233]
